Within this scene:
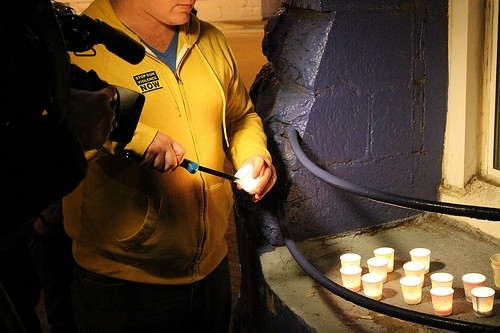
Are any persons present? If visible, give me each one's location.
[63,0,277,333]
[0,0,115,333]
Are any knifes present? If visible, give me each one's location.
[177,156,240,182]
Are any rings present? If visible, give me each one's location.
[271,176,276,185]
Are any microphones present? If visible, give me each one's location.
[74,13,145,65]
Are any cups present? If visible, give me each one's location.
[403,261,426,287]
[340,253,362,268]
[470,287,495,317]
[400,276,424,305]
[490,253,500,268]
[430,273,454,289]
[340,266,362,292]
[430,286,454,316]
[373,247,394,273]
[233,164,262,194]
[361,273,384,301]
[491,262,500,289]
[462,273,486,303]
[367,257,388,284]
[409,248,431,274]
